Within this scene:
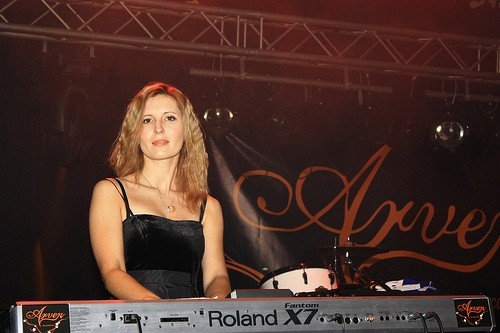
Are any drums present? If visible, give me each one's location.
[258,265,339,296]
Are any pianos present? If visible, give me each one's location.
[8,294,498,333]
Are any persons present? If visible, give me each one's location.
[89,82,233,301]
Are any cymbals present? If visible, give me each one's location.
[302,245,391,254]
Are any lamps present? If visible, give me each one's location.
[203,82,236,130]
[429,106,470,153]
